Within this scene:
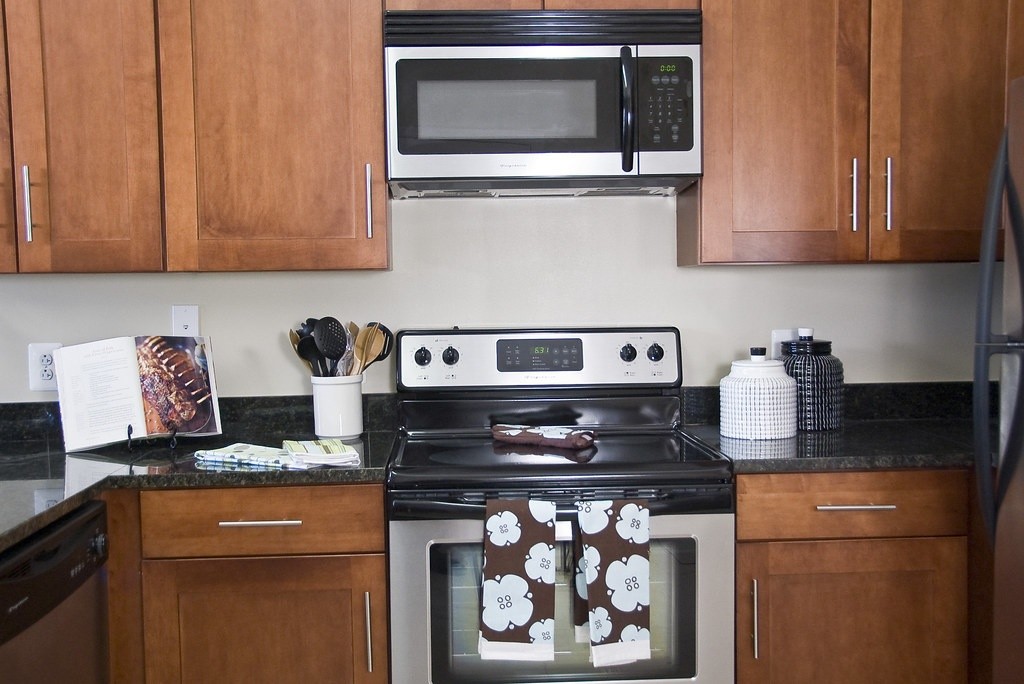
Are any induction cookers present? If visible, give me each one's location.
[392,326,734,489]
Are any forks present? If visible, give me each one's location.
[358,323,381,374]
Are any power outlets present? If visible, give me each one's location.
[28,342,63,391]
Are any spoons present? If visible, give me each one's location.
[290,318,320,376]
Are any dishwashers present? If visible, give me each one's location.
[1,500,111,684]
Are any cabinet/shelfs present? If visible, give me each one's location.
[140,484,389,684]
[158,0,393,272]
[677,0,1008,266]
[5,0,163,275]
[736,469,968,684]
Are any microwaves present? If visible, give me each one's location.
[383,45,702,201]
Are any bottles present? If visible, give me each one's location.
[720,347,798,439]
[779,328,843,431]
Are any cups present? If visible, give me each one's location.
[311,374,363,441]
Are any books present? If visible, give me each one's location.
[52,337,223,454]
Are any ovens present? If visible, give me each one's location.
[386,485,737,684]
